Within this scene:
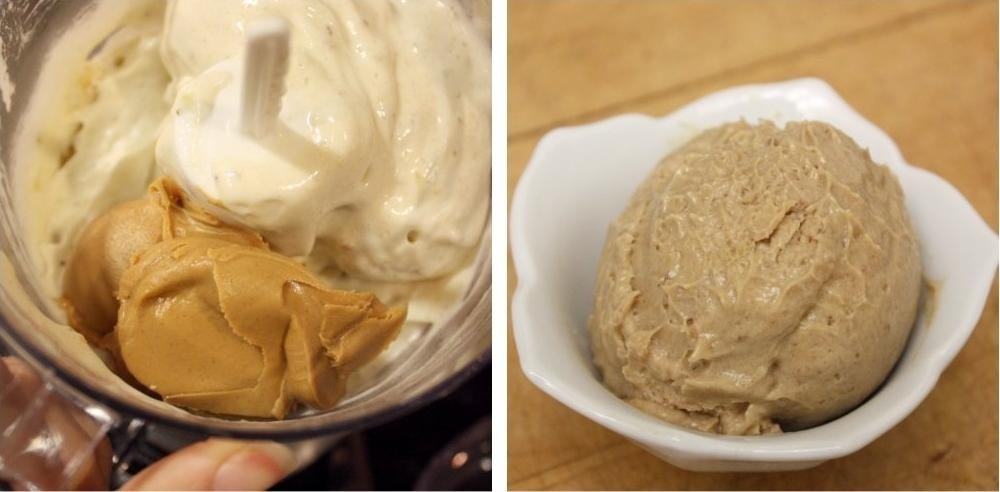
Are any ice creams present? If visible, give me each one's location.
[6,0,492,420]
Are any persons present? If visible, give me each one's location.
[0,346,301,490]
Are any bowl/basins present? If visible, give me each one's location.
[510,76,999,474]
